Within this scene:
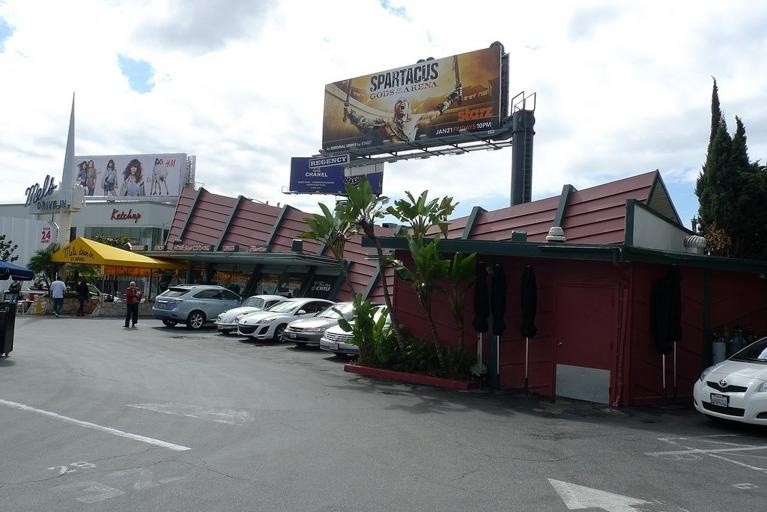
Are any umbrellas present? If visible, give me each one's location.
[0,258,34,281]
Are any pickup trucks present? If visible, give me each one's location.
[26,282,119,303]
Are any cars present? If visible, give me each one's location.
[283,301,364,346]
[320,305,392,356]
[693,337,767,425]
[152,285,246,329]
[4,289,29,312]
[213,295,289,334]
[238,297,336,343]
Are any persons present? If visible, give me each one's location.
[73,157,171,197]
[122,281,141,328]
[74,279,90,317]
[6,279,22,303]
[49,276,67,317]
[343,83,463,142]
